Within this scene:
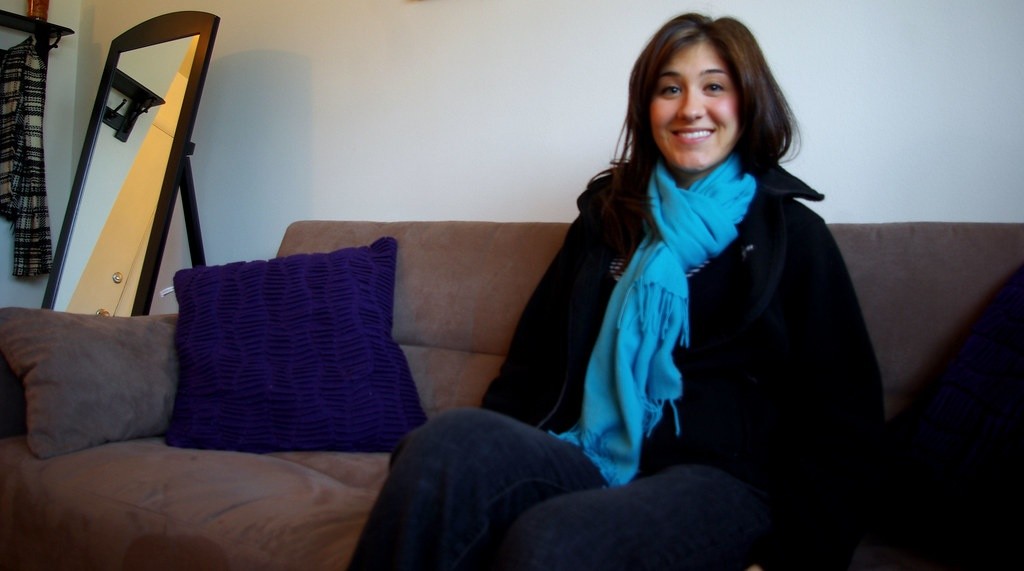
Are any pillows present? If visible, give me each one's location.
[164,233,430,454]
[861,261,1024,571]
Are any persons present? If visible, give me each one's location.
[344,12,886,571]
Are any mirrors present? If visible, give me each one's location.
[41,11,220,320]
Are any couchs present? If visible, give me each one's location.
[0,221,1023,570]
